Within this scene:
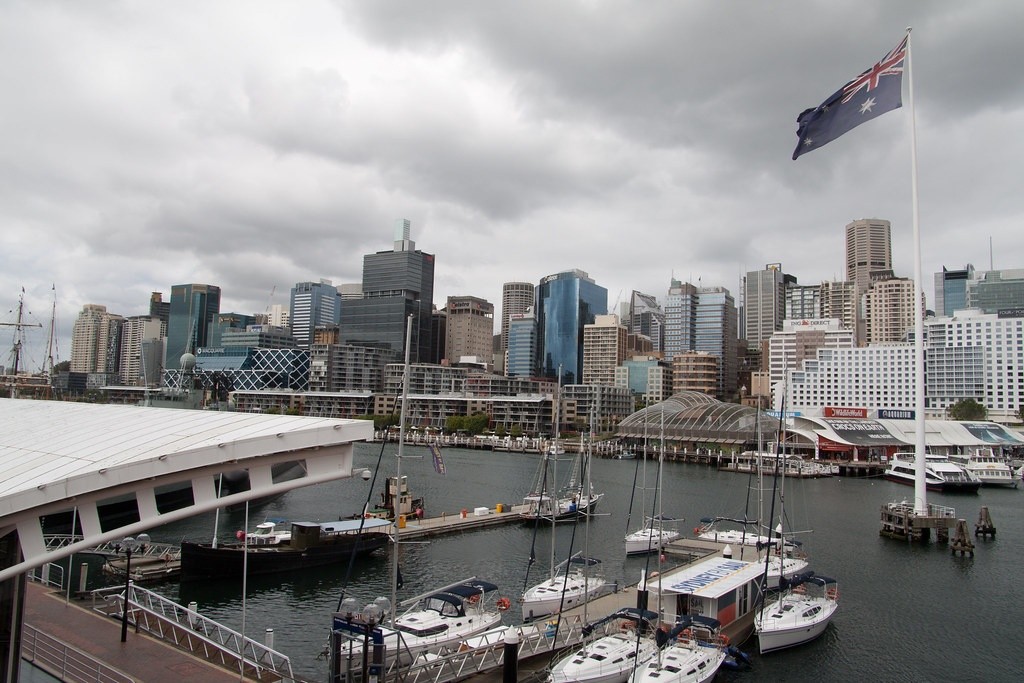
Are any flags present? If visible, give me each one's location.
[427,439,447,475]
[792,34,908,161]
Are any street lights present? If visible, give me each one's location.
[116,533,150,643]
[340,594,393,683]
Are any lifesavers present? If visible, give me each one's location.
[471,593,482,603]
[679,630,692,641]
[622,620,637,633]
[714,633,729,646]
[494,597,510,612]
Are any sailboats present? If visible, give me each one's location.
[321,313,513,675]
[621,381,686,559]
[518,410,605,525]
[538,334,838,682]
[514,361,609,624]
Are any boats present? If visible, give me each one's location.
[177,517,391,587]
[235,520,292,551]
[884,452,985,495]
[947,434,1024,487]
[102,545,183,582]
